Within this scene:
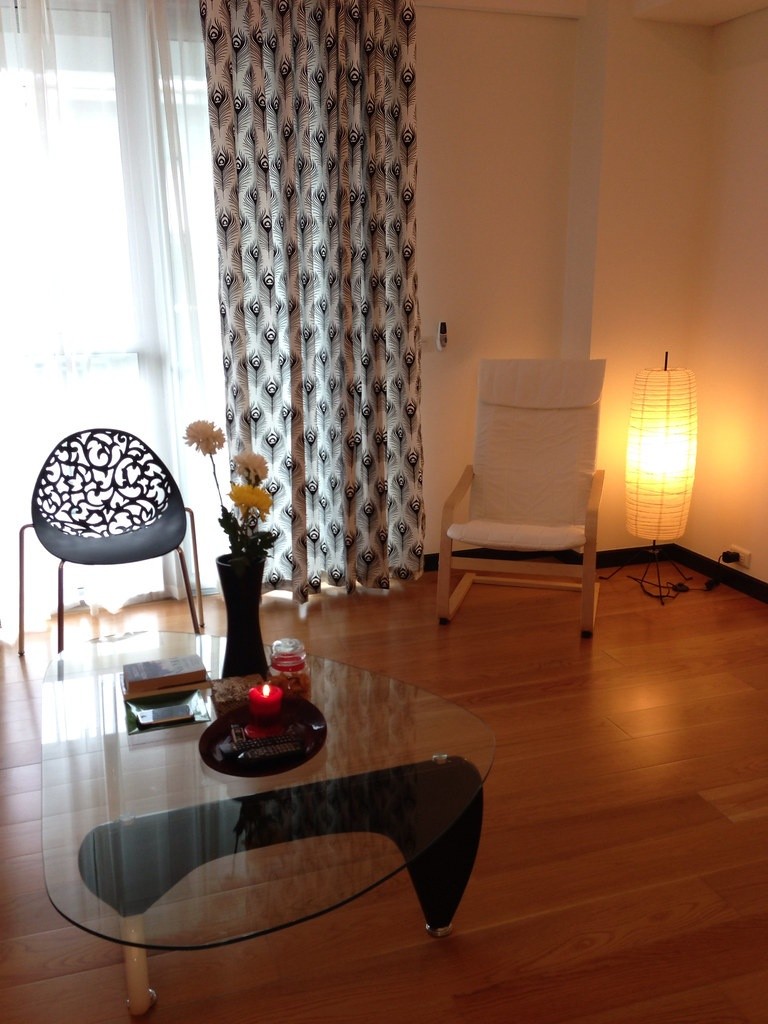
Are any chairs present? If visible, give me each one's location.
[17,427,204,658]
[438,358,607,637]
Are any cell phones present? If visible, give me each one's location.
[137,705,193,727]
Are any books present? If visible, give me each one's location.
[119,654,213,700]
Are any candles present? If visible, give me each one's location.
[248,683,285,717]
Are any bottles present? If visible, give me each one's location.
[267,638,312,703]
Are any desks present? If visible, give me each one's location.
[39,629,498,1016]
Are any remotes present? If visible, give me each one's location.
[218,734,305,762]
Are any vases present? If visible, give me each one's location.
[216,554,266,679]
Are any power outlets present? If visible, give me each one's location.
[731,545,750,569]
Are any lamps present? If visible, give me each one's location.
[599,351,699,605]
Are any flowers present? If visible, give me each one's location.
[183,421,282,560]
